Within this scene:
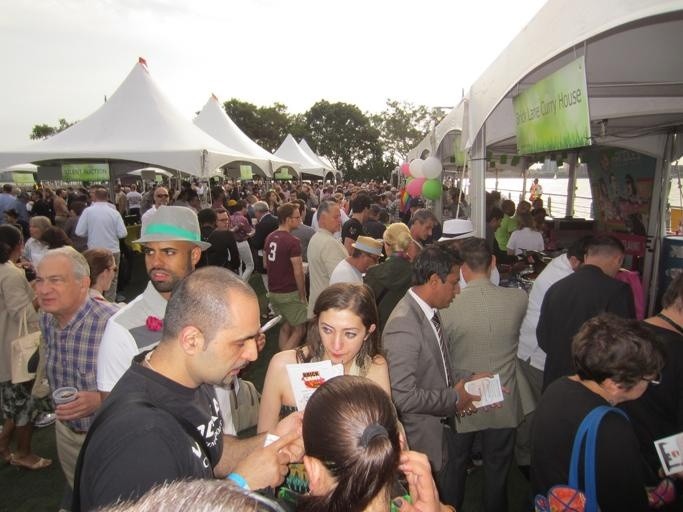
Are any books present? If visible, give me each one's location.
[461,373,504,413]
[285,361,344,414]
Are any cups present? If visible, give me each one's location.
[302,262,308,273]
[52,387,78,425]
[251,218,257,225]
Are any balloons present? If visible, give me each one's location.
[402,158,442,200]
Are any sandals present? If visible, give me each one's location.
[261,311,276,320]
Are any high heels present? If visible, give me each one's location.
[1,431,15,464]
[10,453,54,474]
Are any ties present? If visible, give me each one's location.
[431,313,454,388]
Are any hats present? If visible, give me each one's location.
[131,205,212,252]
[437,218,478,243]
[351,235,386,258]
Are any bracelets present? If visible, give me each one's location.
[226,472,250,493]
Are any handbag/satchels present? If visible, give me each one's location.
[534,404,681,512]
[230,380,261,434]
[10,309,43,385]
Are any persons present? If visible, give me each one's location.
[97,204,266,437]
[486,177,551,254]
[0,224,53,472]
[92,477,280,512]
[438,234,527,509]
[0,174,467,353]
[622,273,682,512]
[378,246,510,511]
[528,313,681,512]
[255,280,411,503]
[295,372,454,511]
[33,242,132,494]
[517,233,637,404]
[71,266,306,512]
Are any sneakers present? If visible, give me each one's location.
[34,411,56,430]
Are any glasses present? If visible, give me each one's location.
[289,216,301,221]
[156,192,170,198]
[610,368,664,387]
[102,266,117,272]
